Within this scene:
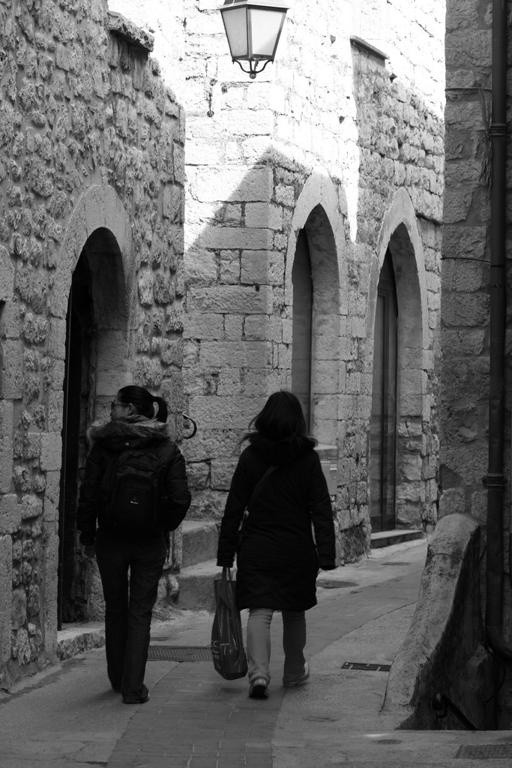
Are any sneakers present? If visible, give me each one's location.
[284,662,310,686]
[249,678,269,700]
[124,684,148,704]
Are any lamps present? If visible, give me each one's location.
[205,0,287,77]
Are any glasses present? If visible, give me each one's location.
[110,400,130,412]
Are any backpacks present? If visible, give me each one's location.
[104,434,165,539]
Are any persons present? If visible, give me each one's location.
[216,391,337,699]
[75,386,192,703]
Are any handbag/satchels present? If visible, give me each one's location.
[211,579,247,680]
